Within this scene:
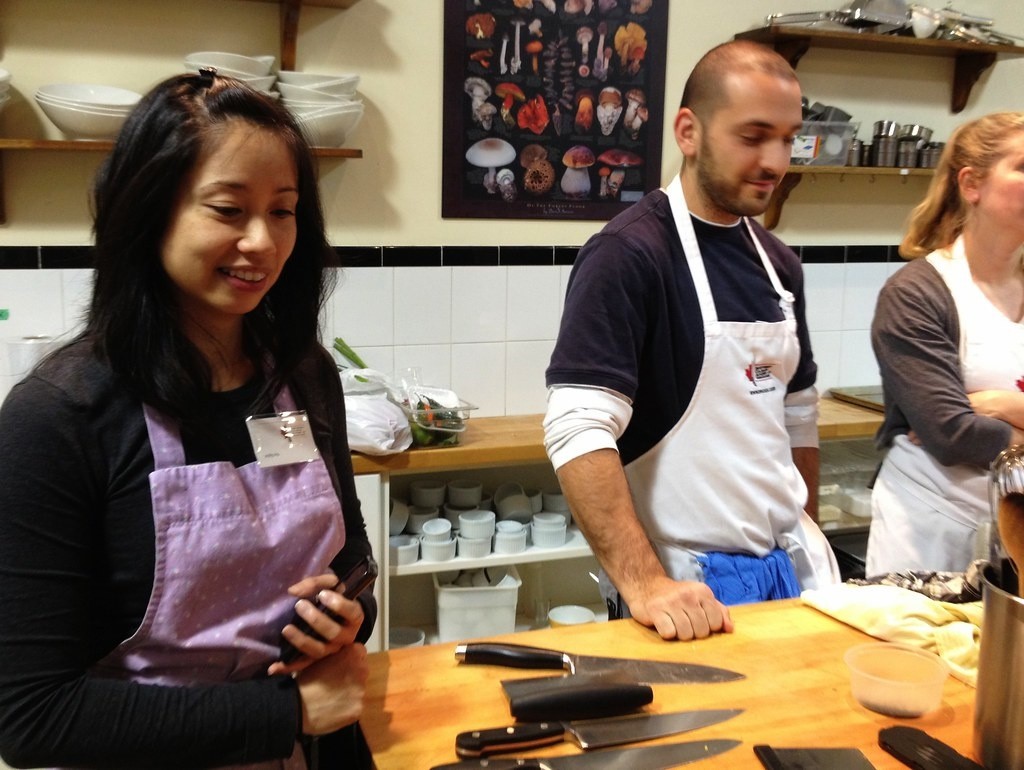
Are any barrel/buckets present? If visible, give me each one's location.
[973,557,1024,770]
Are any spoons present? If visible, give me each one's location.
[998,492,1024,599]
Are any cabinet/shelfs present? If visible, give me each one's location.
[0,0,363,226]
[734,26,1024,232]
[350,397,887,653]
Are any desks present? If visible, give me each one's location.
[360,595,975,770]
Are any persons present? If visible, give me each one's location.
[542,39,842,639]
[868,113,1022,575]
[0,68,380,770]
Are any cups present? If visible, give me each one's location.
[534,597,551,629]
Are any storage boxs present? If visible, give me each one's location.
[790,119,856,167]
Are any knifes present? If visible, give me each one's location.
[454,643,747,684]
[454,709,753,758]
[426,739,744,770]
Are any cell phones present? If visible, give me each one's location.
[280,558,379,665]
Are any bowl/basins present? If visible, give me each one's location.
[389,627,425,648]
[389,473,572,565]
[438,566,517,587]
[802,97,851,137]
[33,84,143,141]
[844,642,948,718]
[183,51,278,106]
[1,70,13,112]
[548,605,595,628]
[278,70,364,146]
[847,120,946,169]
